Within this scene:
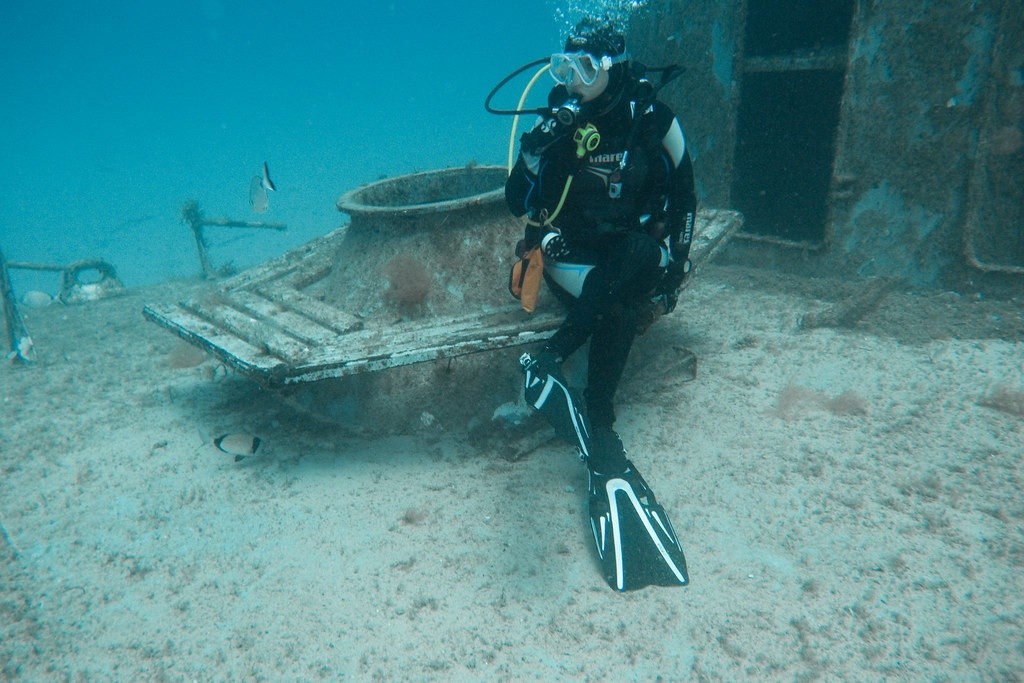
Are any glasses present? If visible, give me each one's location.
[549,50,613,85]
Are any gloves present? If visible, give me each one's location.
[522,115,572,155]
[654,266,684,296]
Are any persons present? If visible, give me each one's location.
[505,14,695,476]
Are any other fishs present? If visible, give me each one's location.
[213,431,270,462]
[249,160,276,212]
[21,291,68,311]
